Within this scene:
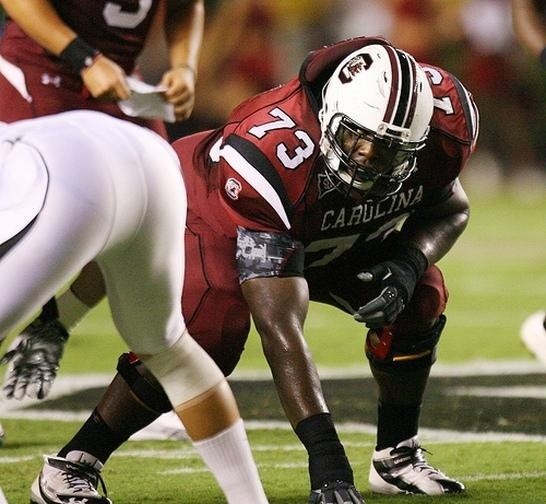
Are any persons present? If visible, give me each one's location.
[0,109,270,504]
[31,37,479,504]
[190,1,546,197]
[1,0,204,401]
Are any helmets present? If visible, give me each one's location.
[318,44,435,207]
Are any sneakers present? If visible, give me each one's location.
[368,435,467,495]
[29,450,113,504]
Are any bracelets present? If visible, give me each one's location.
[173,63,199,85]
[60,35,99,72]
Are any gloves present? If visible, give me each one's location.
[1,309,69,399]
[353,257,417,331]
[309,443,366,504]
